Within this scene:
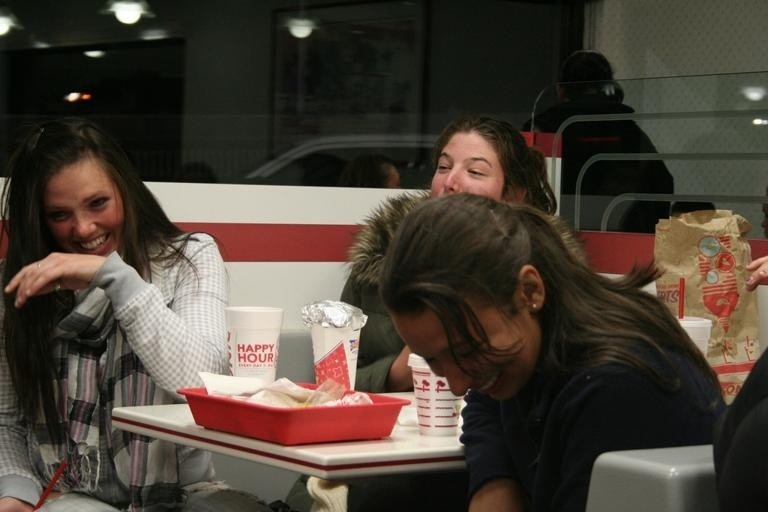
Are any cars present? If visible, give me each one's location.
[244,129,471,195]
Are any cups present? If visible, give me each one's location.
[311,301,363,391]
[406,352,462,438]
[224,305,284,382]
[675,315,713,358]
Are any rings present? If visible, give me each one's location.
[53,280,60,291]
[36,258,42,270]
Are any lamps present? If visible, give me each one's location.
[280,1,319,40]
[100,0,156,25]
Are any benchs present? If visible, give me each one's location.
[0,177,434,504]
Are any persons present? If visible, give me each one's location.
[1,114,274,512]
[336,152,402,190]
[521,49,674,231]
[741,255,767,291]
[282,110,559,510]
[379,189,726,511]
